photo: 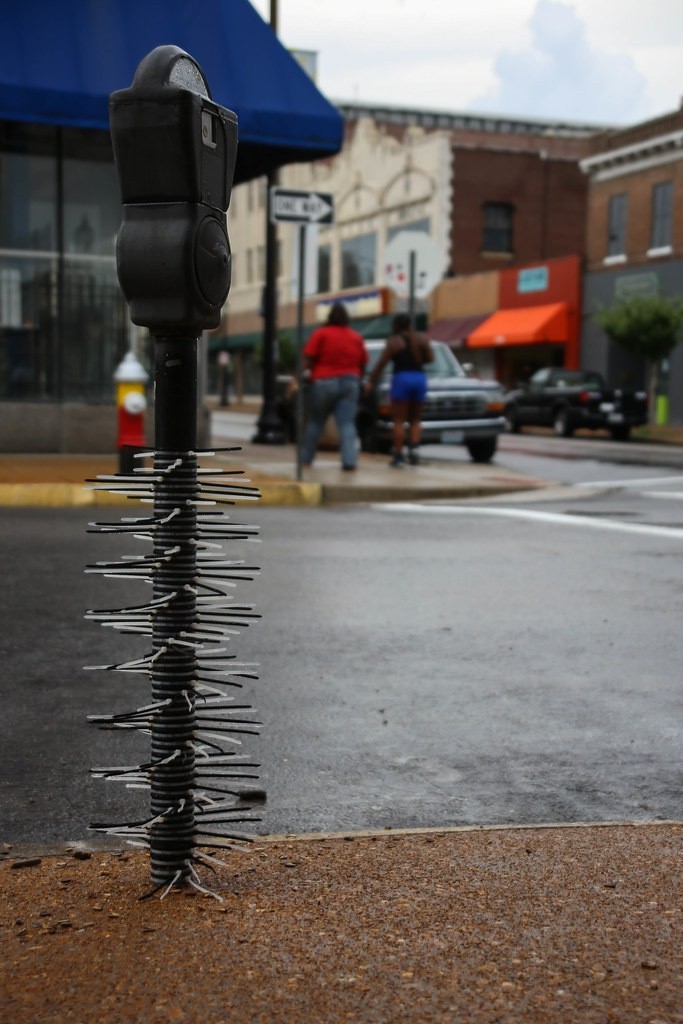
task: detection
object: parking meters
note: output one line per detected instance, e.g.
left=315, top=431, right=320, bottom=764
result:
left=109, top=43, right=241, bottom=893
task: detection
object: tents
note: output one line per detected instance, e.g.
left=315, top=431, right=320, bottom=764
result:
left=0, top=0, right=346, bottom=405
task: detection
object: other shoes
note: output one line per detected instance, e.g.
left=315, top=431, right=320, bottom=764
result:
left=341, top=467, right=356, bottom=482
left=409, top=451, right=421, bottom=464
left=300, top=464, right=312, bottom=481
left=389, top=455, right=405, bottom=469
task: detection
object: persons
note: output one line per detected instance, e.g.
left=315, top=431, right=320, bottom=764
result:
left=298, top=303, right=370, bottom=471
left=362, top=312, right=434, bottom=466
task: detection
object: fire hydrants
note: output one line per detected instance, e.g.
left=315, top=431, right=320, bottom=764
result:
left=112, top=350, right=151, bottom=478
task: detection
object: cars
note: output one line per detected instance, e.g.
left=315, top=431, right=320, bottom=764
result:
left=498, top=367, right=649, bottom=440
left=277, top=339, right=509, bottom=463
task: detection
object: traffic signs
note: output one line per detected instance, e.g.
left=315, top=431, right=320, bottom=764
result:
left=271, top=184, right=335, bottom=224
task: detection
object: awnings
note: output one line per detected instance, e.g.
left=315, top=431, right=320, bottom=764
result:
left=466, top=300, right=568, bottom=347
left=426, top=310, right=492, bottom=348
